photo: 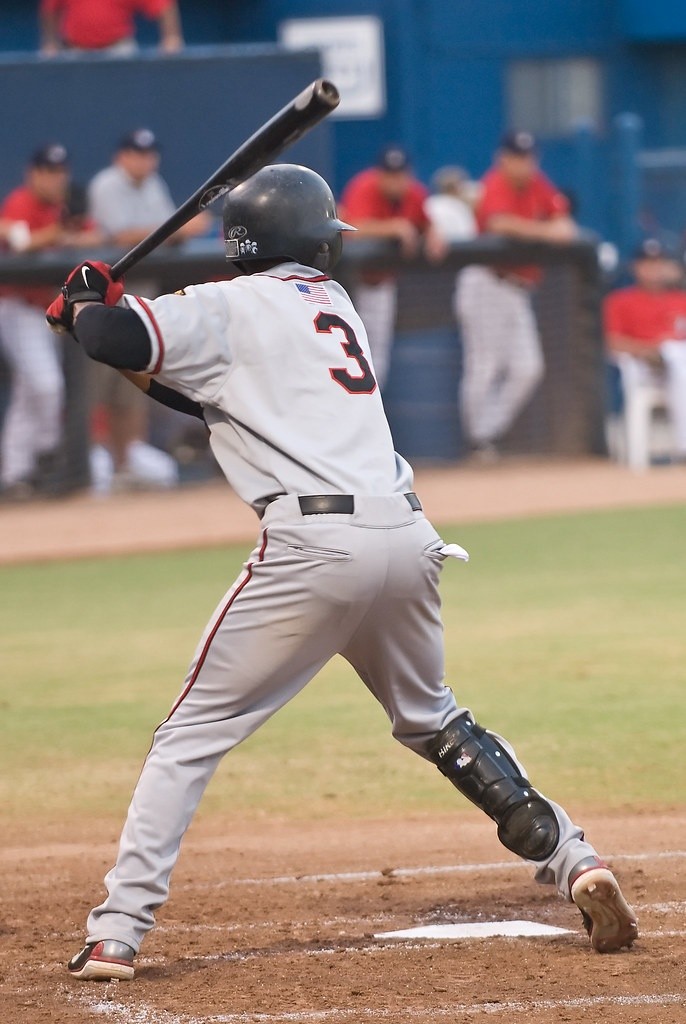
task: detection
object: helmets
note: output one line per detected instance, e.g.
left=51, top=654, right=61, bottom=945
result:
left=222, top=164, right=359, bottom=275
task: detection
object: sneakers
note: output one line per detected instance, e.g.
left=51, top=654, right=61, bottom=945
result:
left=68, top=939, right=135, bottom=980
left=568, top=855, right=638, bottom=953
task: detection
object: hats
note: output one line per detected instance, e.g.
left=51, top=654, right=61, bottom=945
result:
left=382, top=145, right=410, bottom=171
left=498, top=128, right=541, bottom=154
left=35, top=142, right=74, bottom=171
left=118, top=128, right=163, bottom=151
left=634, top=235, right=678, bottom=263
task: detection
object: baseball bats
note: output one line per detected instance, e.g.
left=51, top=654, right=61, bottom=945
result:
left=109, top=77, right=341, bottom=282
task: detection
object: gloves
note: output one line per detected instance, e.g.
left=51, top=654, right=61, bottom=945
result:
left=62, top=260, right=125, bottom=317
left=46, top=293, right=68, bottom=335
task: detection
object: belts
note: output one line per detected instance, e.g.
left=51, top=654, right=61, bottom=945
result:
left=298, top=492, right=423, bottom=516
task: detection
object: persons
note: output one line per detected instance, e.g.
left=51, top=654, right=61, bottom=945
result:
left=336, top=150, right=430, bottom=394
left=422, top=166, right=480, bottom=241
left=84, top=130, right=212, bottom=497
left=37, top=0, right=187, bottom=59
left=44, top=162, right=639, bottom=980
left=602, top=236, right=686, bottom=471
left=428, top=132, right=577, bottom=472
left=0, top=148, right=108, bottom=503
